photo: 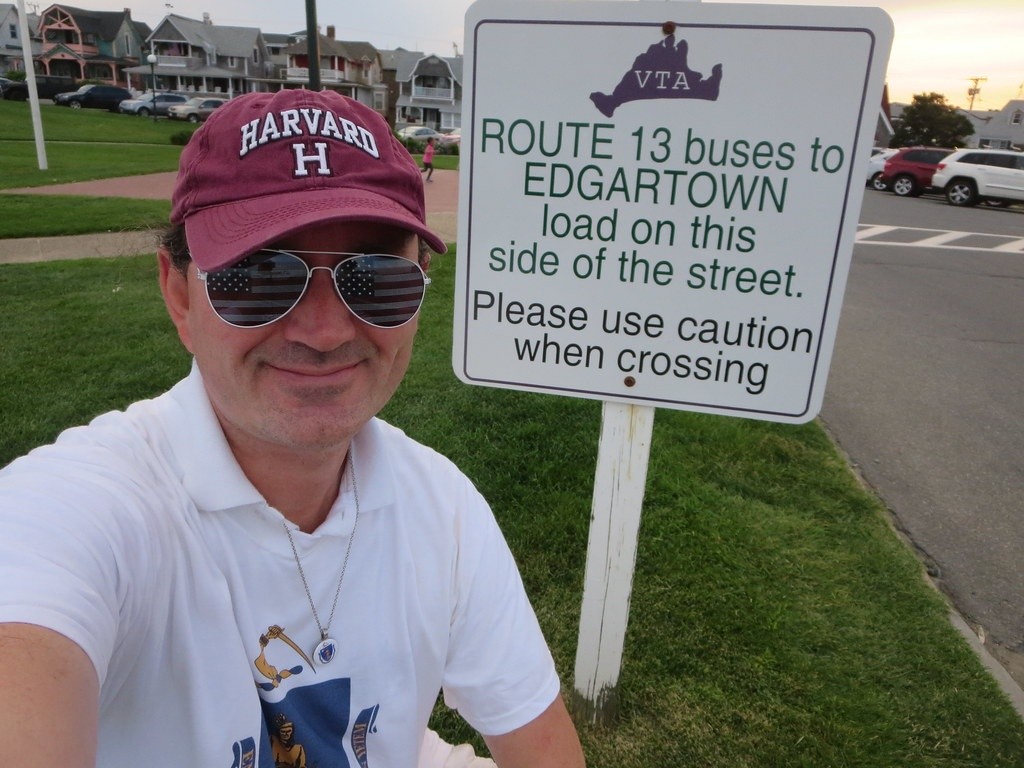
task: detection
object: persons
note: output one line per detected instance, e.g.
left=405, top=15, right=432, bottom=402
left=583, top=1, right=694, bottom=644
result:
left=420, top=137, right=436, bottom=182
left=2, top=91, right=589, bottom=768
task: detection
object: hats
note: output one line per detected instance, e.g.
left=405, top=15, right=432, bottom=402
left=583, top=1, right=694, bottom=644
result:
left=170, top=86, right=447, bottom=272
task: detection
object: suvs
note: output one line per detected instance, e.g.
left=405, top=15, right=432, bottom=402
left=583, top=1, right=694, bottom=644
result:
left=883, top=147, right=952, bottom=199
left=5, top=72, right=75, bottom=104
left=932, top=146, right=1024, bottom=210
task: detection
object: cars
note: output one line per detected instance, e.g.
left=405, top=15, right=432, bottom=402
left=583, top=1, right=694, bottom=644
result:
left=866, top=147, right=899, bottom=193
left=440, top=126, right=461, bottom=149
left=119, top=91, right=191, bottom=119
left=167, top=94, right=227, bottom=123
left=0, top=77, right=11, bottom=93
left=396, top=121, right=441, bottom=142
left=51, top=83, right=129, bottom=110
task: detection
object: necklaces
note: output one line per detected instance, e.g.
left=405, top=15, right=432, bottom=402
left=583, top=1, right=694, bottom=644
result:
left=277, top=445, right=360, bottom=663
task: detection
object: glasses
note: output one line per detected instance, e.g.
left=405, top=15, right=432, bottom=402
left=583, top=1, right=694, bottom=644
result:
left=196, top=248, right=432, bottom=329
